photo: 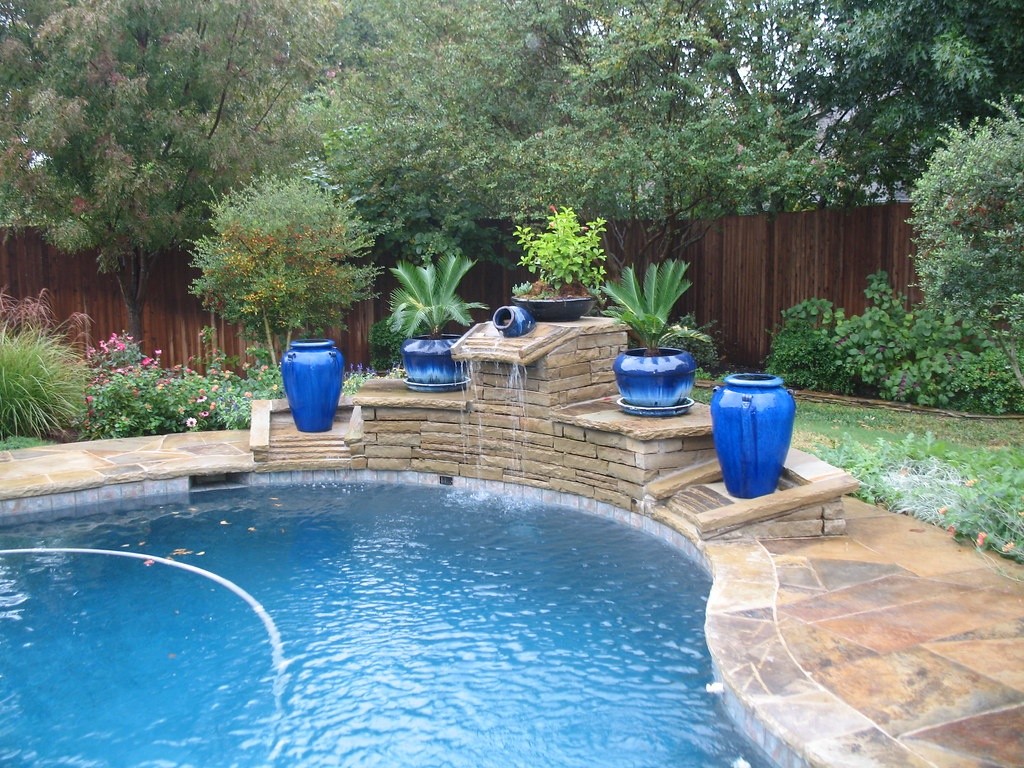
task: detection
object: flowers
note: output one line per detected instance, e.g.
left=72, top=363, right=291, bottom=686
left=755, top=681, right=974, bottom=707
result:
left=509, top=205, right=607, bottom=287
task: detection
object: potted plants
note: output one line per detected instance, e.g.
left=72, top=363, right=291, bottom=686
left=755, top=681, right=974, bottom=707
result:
left=510, top=281, right=597, bottom=322
left=596, top=260, right=711, bottom=419
left=386, top=253, right=472, bottom=392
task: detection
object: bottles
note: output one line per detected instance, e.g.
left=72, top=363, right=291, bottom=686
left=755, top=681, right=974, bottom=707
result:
left=492, top=305, right=534, bottom=338
left=281, top=338, right=345, bottom=433
left=710, top=373, right=796, bottom=499
